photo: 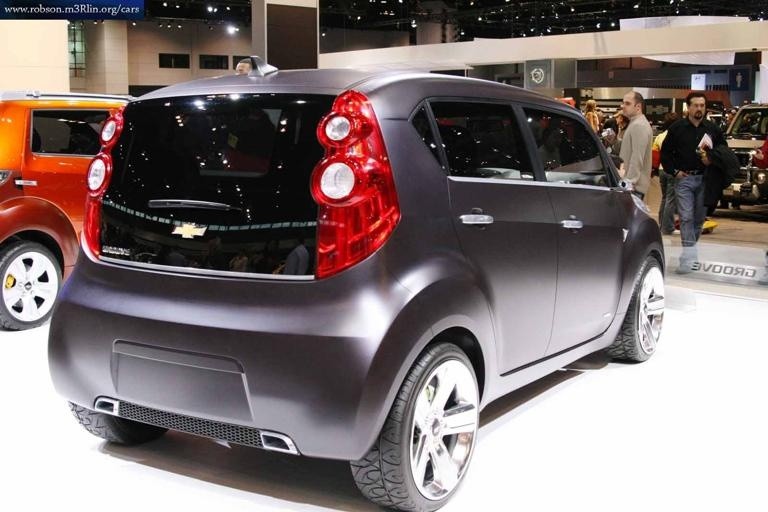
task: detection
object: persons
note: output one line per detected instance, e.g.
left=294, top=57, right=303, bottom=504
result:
left=100, top=221, right=312, bottom=276
left=233, top=56, right=253, bottom=77
left=538, top=125, right=565, bottom=169
left=581, top=89, right=757, bottom=276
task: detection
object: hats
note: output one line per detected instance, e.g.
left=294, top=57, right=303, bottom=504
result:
left=613, top=111, right=630, bottom=120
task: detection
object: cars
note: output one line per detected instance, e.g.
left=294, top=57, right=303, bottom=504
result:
left=1, top=97, right=278, bottom=330
left=48, top=54, right=668, bottom=512
left=706, top=114, right=728, bottom=128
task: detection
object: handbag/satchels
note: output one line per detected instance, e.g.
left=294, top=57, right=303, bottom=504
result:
left=651, top=144, right=661, bottom=170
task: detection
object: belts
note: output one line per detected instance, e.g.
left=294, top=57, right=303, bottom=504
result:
left=686, top=169, right=704, bottom=176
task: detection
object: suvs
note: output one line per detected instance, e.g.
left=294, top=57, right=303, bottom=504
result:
left=718, top=99, right=768, bottom=209
